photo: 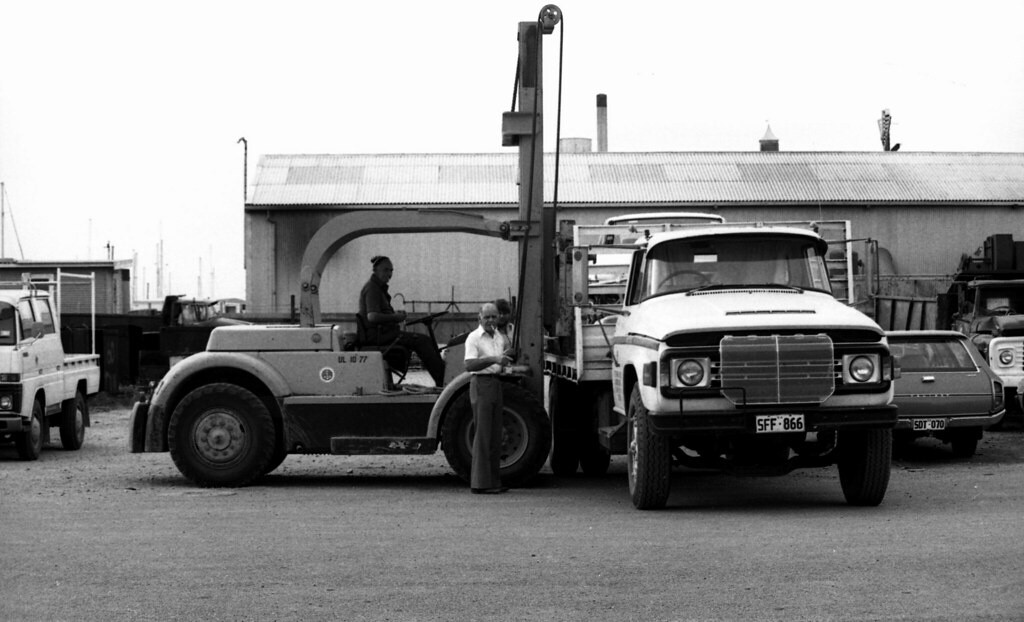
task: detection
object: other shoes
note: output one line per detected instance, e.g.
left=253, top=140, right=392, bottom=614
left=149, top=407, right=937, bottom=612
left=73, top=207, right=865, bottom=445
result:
left=471, top=486, right=511, bottom=494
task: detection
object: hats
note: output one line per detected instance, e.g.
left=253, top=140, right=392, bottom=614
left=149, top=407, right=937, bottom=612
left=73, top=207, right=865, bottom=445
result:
left=495, top=299, right=511, bottom=314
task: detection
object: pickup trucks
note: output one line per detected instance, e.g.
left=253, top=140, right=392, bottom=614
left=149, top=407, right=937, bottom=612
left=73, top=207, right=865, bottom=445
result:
left=0, top=288, right=103, bottom=459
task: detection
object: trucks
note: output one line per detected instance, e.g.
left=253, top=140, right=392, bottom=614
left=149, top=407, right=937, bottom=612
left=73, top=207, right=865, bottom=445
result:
left=550, top=209, right=902, bottom=508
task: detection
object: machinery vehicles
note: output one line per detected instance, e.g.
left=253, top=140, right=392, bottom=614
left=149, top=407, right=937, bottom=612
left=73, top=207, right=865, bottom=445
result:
left=129, top=3, right=559, bottom=490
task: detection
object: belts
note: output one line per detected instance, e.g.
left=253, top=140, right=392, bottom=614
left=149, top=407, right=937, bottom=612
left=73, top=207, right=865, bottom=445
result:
left=472, top=373, right=500, bottom=378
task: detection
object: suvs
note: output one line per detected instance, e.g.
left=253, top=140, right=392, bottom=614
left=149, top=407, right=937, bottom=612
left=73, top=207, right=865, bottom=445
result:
left=950, top=278, right=1024, bottom=429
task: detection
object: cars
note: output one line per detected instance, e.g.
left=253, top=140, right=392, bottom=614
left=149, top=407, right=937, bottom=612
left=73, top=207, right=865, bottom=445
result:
left=881, top=327, right=1007, bottom=460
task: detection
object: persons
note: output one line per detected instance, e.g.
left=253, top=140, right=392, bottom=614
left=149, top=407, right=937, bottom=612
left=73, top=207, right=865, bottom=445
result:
left=950, top=300, right=975, bottom=337
left=464, top=299, right=516, bottom=494
left=358, top=255, right=447, bottom=386
